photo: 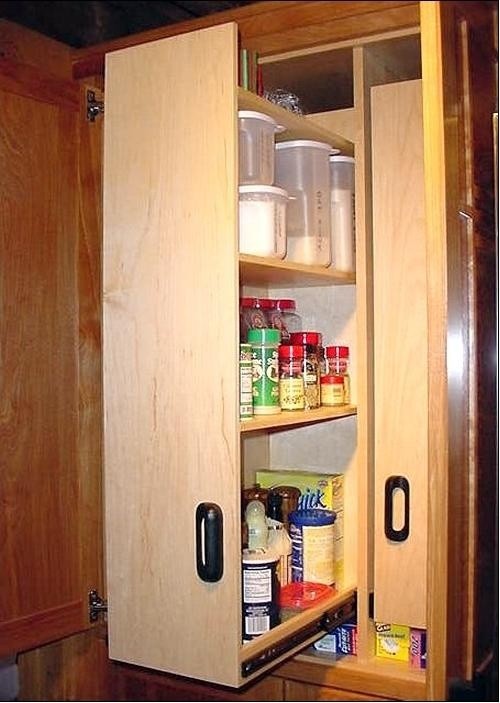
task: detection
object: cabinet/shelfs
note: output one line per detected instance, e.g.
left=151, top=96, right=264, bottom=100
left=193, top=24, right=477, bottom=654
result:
left=0, top=0, right=499, bottom=702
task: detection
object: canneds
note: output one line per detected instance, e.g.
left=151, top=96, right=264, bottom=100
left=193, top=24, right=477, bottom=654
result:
left=240, top=297, right=350, bottom=419
left=287, top=508, right=337, bottom=588
left=242, top=554, right=280, bottom=641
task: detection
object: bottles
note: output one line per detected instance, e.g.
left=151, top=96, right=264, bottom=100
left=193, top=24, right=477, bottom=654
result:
left=243, top=492, right=283, bottom=553
left=241, top=298, right=352, bottom=420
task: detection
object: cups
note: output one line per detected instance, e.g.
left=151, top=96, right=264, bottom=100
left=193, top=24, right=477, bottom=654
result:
left=239, top=110, right=356, bottom=273
